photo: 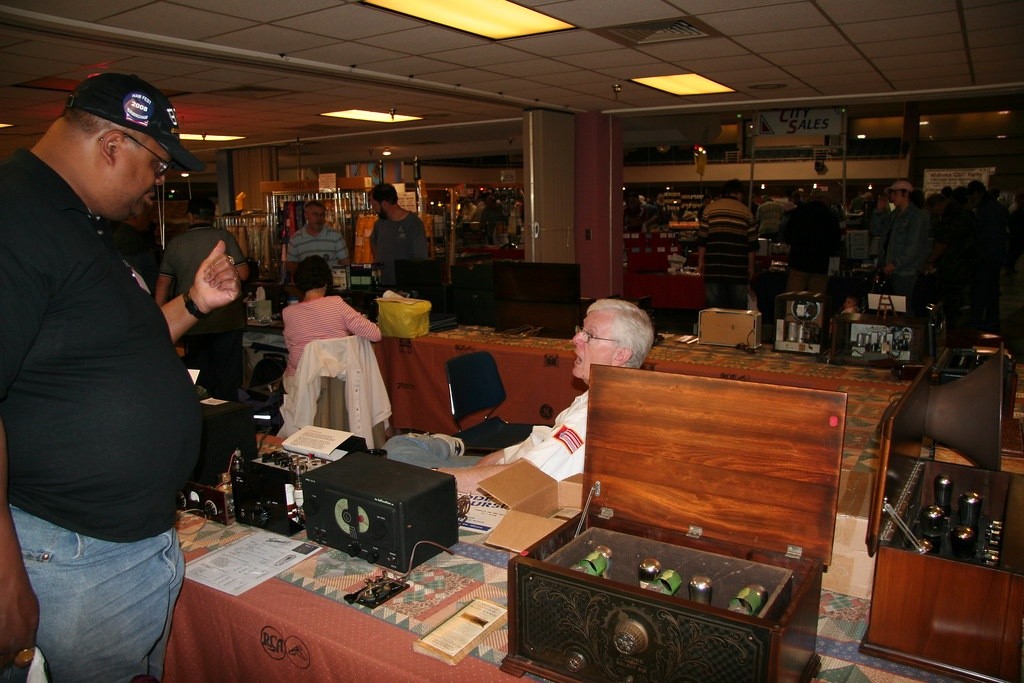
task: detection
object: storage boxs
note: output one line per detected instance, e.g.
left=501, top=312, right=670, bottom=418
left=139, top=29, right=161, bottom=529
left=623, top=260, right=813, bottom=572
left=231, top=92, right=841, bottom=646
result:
left=866, top=357, right=1024, bottom=683
left=476, top=461, right=584, bottom=559
left=259, top=176, right=372, bottom=193
left=498, top=364, right=849, bottom=683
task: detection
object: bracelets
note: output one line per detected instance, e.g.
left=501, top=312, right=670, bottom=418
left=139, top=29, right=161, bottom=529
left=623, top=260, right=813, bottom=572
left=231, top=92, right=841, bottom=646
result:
left=430, top=466, right=438, bottom=471
left=181, top=290, right=212, bottom=320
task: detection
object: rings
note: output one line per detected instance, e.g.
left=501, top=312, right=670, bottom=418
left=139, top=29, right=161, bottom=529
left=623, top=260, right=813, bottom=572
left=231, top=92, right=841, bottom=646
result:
left=233, top=268, right=240, bottom=283
left=228, top=255, right=235, bottom=265
left=14, top=647, right=34, bottom=669
left=0, top=72, right=240, bottom=683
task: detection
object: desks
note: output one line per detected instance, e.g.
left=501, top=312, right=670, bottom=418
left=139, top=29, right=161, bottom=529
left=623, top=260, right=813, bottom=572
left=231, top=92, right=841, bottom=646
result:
left=277, top=336, right=394, bottom=450
left=623, top=268, right=706, bottom=309
left=162, top=324, right=1024, bottom=682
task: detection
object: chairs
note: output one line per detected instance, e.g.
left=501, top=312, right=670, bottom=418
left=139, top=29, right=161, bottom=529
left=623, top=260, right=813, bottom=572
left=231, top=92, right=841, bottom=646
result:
left=443, top=350, right=553, bottom=456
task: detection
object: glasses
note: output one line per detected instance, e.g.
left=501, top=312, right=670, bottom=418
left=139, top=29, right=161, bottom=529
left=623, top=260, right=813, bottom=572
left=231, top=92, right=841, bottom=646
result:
left=98, top=133, right=173, bottom=177
left=575, top=325, right=616, bottom=343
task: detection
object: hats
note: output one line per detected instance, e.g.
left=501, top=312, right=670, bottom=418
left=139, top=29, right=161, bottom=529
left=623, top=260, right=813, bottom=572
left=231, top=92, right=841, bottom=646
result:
left=887, top=181, right=913, bottom=192
left=182, top=197, right=215, bottom=219
left=65, top=74, right=208, bottom=171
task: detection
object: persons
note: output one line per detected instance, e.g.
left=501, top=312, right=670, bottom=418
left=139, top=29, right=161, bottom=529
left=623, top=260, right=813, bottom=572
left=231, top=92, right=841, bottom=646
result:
left=381, top=298, right=654, bottom=496
left=368, top=183, right=430, bottom=285
left=111, top=193, right=249, bottom=398
left=695, top=179, right=1024, bottom=368
left=623, top=193, right=697, bottom=231
left=275, top=255, right=382, bottom=413
left=285, top=200, right=350, bottom=297
left=458, top=192, right=524, bottom=245
